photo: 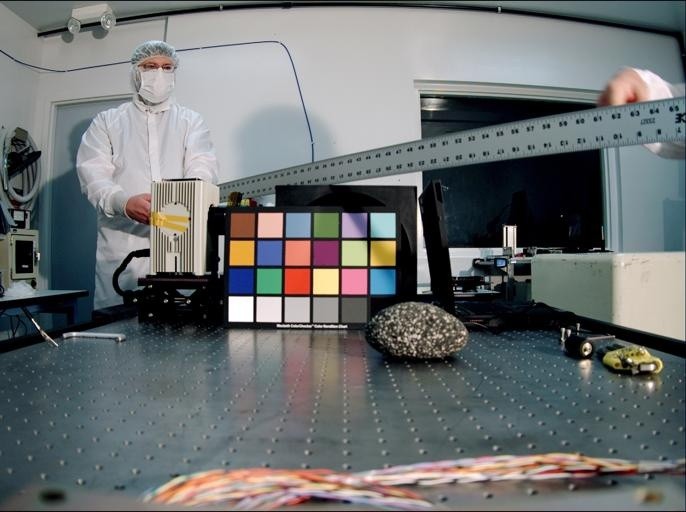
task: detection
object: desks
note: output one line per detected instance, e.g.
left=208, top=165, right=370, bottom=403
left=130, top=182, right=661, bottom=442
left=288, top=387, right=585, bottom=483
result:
left=0, top=290, right=89, bottom=326
left=474, top=256, right=531, bottom=304
left=417, top=286, right=502, bottom=300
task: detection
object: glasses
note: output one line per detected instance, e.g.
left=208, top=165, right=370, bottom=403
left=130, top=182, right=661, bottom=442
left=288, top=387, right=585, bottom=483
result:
left=138, top=63, right=174, bottom=72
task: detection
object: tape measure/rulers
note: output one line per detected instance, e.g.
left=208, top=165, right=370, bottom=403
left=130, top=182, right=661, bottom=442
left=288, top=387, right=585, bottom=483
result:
left=218, top=97, right=686, bottom=203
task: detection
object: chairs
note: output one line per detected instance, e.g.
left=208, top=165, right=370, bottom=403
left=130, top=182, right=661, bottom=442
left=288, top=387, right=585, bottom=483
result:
left=418, top=178, right=575, bottom=331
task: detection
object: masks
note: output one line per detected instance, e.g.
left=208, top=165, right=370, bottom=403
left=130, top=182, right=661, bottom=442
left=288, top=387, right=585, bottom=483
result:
left=138, top=69, right=174, bottom=104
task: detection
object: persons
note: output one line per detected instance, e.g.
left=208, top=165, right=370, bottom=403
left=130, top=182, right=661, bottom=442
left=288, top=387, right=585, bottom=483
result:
left=77, top=40, right=220, bottom=309
left=597, top=65, right=685, bottom=159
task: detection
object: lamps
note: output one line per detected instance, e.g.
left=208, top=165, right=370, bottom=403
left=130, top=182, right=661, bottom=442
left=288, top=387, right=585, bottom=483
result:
left=67, top=4, right=116, bottom=34
